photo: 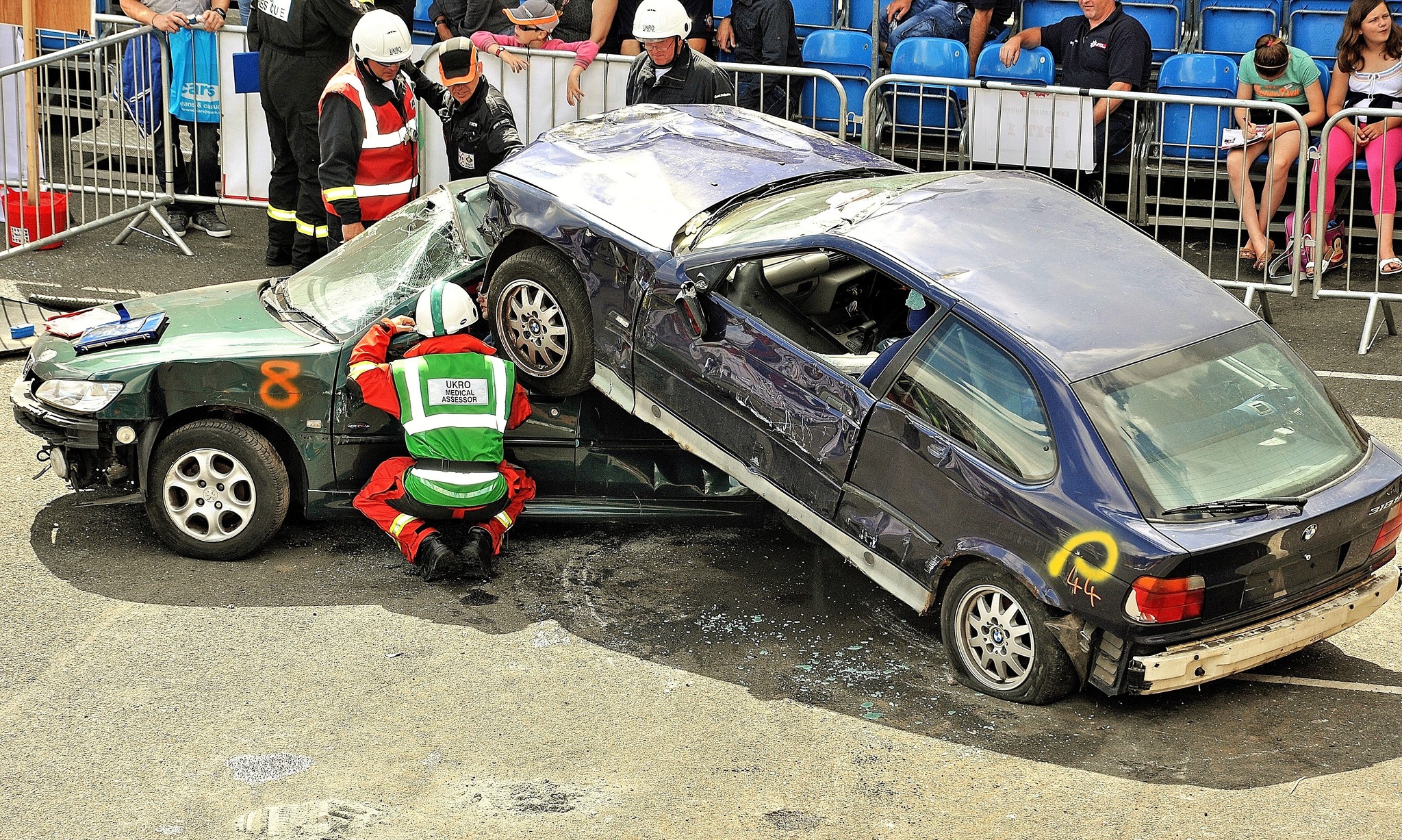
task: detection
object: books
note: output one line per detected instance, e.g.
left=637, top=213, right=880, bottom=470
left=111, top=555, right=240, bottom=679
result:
left=233, top=52, right=261, bottom=94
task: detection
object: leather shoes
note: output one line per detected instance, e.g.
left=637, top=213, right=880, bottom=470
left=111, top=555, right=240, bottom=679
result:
left=459, top=527, right=491, bottom=580
left=418, top=536, right=454, bottom=583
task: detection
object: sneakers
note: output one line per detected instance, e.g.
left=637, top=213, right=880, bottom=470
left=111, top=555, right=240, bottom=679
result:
left=189, top=210, right=232, bottom=237
left=162, top=212, right=189, bottom=235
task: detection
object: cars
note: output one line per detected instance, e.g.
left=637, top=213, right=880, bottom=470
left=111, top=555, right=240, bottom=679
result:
left=485, top=99, right=1402, bottom=706
left=10, top=173, right=827, bottom=560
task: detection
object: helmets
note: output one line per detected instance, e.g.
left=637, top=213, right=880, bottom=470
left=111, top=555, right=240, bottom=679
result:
left=351, top=7, right=411, bottom=62
left=632, top=0, right=693, bottom=41
left=416, top=280, right=477, bottom=338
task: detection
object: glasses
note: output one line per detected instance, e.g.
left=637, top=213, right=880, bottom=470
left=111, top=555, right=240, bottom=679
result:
left=639, top=38, right=675, bottom=52
left=378, top=57, right=410, bottom=67
left=518, top=24, right=541, bottom=31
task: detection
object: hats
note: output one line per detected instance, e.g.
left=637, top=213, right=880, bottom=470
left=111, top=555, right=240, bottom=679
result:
left=437, top=35, right=477, bottom=87
left=503, top=0, right=560, bottom=33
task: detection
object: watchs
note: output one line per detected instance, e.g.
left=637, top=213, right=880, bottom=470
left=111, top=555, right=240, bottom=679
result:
left=495, top=46, right=505, bottom=58
left=434, top=20, right=445, bottom=27
left=213, top=8, right=226, bottom=21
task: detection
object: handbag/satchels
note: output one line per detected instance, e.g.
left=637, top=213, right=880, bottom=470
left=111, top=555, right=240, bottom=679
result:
left=1267, top=207, right=1350, bottom=283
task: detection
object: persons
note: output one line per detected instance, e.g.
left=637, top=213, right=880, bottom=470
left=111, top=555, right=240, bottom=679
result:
left=866, top=0, right=1013, bottom=82
left=374, top=0, right=417, bottom=36
left=476, top=277, right=496, bottom=347
left=428, top=0, right=520, bottom=51
left=1226, top=34, right=1326, bottom=268
left=246, top=1, right=376, bottom=276
left=626, top=0, right=737, bottom=109
left=716, top=0, right=805, bottom=120
left=999, top=0, right=1152, bottom=208
left=317, top=10, right=418, bottom=250
left=549, top=0, right=618, bottom=52
left=620, top=0, right=714, bottom=62
left=1306, top=0, right=1402, bottom=281
left=470, top=0, right=599, bottom=106
left=400, top=36, right=525, bottom=182
left=347, top=281, right=536, bottom=583
left=113, top=0, right=233, bottom=238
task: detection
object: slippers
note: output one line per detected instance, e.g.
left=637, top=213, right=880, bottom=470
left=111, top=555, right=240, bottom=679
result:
left=1306, top=243, right=1335, bottom=281
left=1377, top=258, right=1402, bottom=274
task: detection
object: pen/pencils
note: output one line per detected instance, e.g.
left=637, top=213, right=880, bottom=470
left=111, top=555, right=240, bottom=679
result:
left=1242, top=116, right=1258, bottom=138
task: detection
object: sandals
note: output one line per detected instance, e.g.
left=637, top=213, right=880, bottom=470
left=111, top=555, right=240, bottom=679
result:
left=1238, top=245, right=1258, bottom=262
left=1253, top=235, right=1275, bottom=270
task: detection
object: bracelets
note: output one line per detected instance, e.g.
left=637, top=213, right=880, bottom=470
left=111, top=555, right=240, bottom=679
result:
left=151, top=13, right=161, bottom=26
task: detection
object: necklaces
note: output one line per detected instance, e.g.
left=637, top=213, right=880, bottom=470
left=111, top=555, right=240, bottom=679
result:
left=556, top=0, right=571, bottom=16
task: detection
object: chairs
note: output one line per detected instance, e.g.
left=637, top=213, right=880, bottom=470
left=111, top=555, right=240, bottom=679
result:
left=414, top=0, right=1402, bottom=167
